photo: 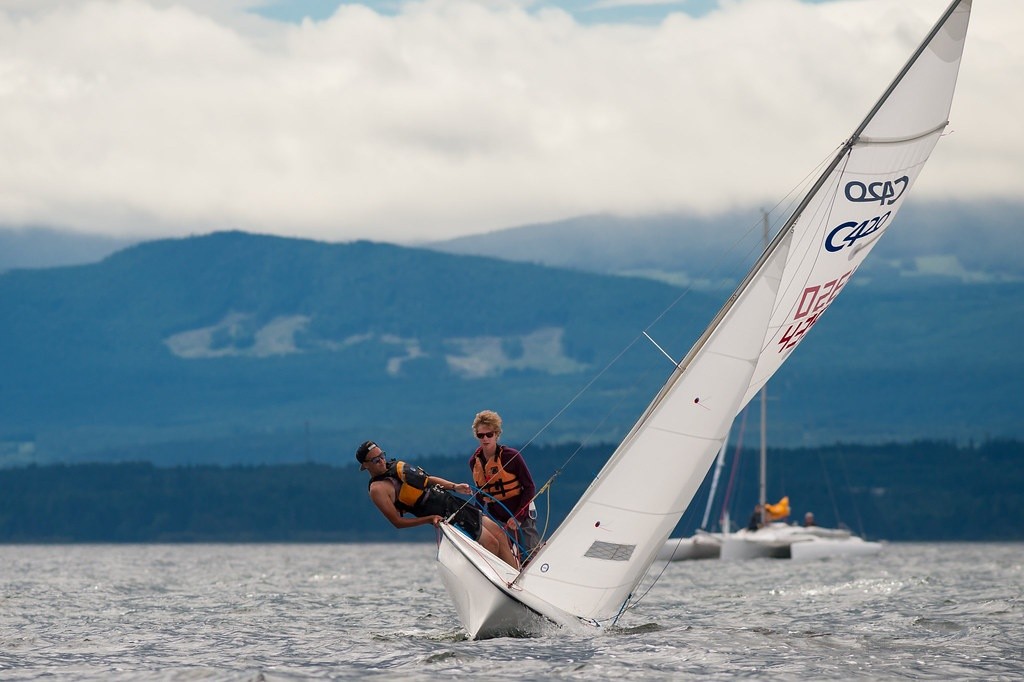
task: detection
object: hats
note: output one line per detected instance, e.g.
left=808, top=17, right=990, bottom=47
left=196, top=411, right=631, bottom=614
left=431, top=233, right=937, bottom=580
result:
left=355, top=441, right=378, bottom=471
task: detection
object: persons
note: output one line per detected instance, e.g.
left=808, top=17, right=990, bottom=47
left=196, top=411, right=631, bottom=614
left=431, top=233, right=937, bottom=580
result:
left=470, top=410, right=541, bottom=571
left=802, top=513, right=816, bottom=528
left=357, top=441, right=519, bottom=572
left=748, top=504, right=763, bottom=532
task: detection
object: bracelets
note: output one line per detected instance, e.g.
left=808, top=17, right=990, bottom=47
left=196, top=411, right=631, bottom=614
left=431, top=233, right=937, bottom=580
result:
left=452, top=483, right=456, bottom=492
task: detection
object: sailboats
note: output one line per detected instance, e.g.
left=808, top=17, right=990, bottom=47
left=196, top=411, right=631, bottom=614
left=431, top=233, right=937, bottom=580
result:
left=646, top=205, right=884, bottom=562
left=432, top=0, right=970, bottom=647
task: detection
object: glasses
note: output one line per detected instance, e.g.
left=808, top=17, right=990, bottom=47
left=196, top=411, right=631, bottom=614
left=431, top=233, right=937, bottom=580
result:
left=363, top=451, right=386, bottom=464
left=477, top=431, right=495, bottom=439
